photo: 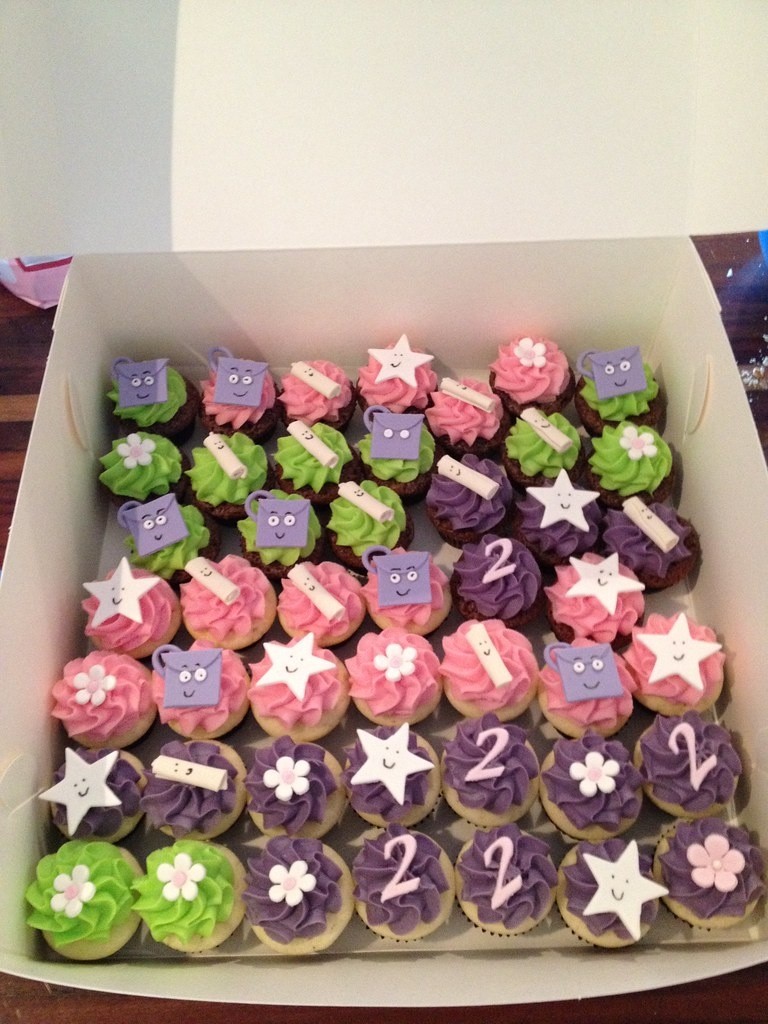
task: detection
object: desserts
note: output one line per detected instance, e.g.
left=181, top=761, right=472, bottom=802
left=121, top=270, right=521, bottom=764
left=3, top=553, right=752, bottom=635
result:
left=25, top=334, right=768, bottom=948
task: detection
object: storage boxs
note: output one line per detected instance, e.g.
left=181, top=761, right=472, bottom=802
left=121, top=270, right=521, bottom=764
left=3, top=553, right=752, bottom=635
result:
left=0, top=232, right=767, bottom=1022
